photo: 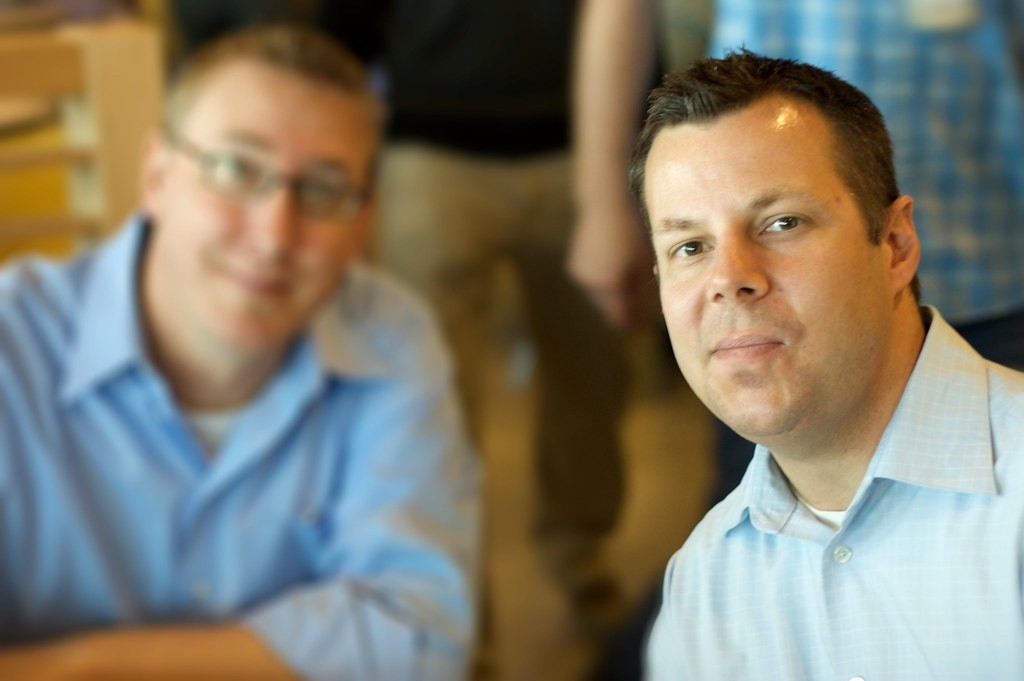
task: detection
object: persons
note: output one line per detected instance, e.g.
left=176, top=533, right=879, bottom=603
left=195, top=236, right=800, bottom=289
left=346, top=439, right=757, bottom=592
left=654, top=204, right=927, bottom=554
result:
left=163, top=0, right=1024, bottom=681
left=0, top=27, right=487, bottom=681
left=631, top=51, right=1024, bottom=681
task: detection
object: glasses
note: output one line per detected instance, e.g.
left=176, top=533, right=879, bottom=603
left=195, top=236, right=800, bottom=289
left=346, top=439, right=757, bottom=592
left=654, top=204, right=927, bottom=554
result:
left=164, top=131, right=370, bottom=219
